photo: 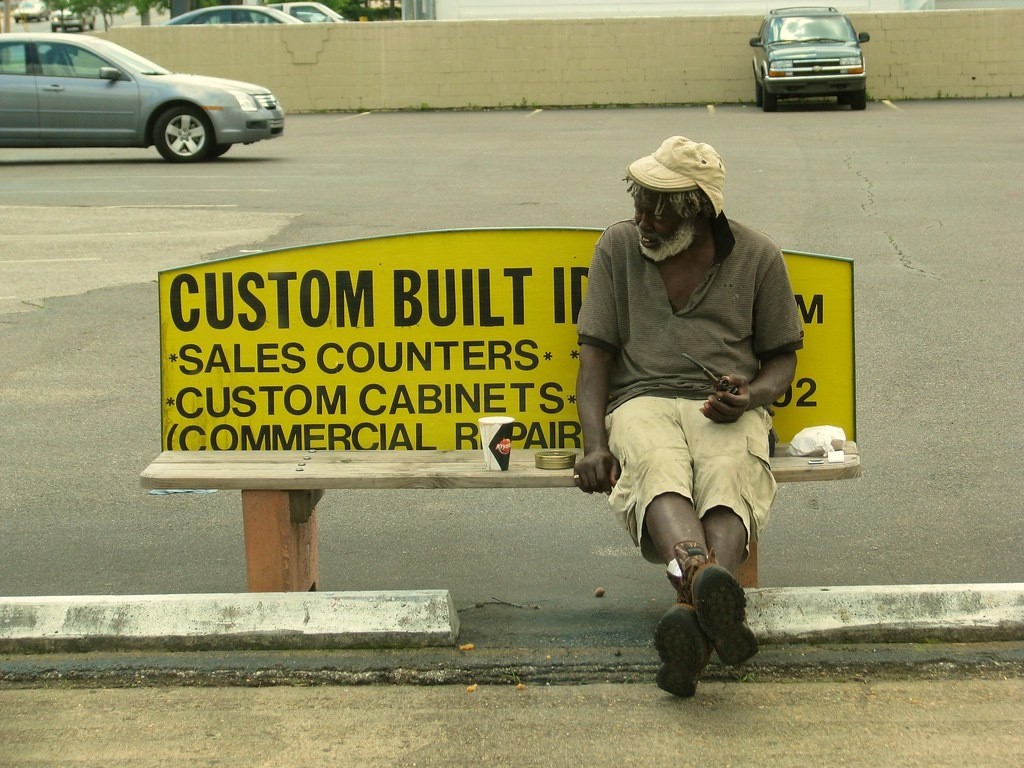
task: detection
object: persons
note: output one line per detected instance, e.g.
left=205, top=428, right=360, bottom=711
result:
left=573, top=135, right=804, bottom=699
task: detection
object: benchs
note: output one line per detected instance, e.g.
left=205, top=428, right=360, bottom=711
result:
left=140, top=228, right=863, bottom=593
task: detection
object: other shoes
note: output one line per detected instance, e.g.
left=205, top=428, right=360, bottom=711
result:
left=654, top=540, right=759, bottom=697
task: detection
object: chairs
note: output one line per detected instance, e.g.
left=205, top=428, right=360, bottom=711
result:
left=39, top=50, right=69, bottom=76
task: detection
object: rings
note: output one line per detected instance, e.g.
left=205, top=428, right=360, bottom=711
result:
left=573, top=474, right=579, bottom=478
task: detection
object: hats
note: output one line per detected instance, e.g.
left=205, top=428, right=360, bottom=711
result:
left=629, top=135, right=726, bottom=220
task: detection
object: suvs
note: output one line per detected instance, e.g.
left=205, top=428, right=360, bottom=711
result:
left=50, top=2, right=95, bottom=32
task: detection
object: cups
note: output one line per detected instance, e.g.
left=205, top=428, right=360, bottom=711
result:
left=478, top=417, right=515, bottom=471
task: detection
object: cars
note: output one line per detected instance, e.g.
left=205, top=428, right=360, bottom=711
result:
left=748, top=6, right=870, bottom=112
left=0, top=32, right=284, bottom=163
left=162, top=5, right=304, bottom=26
left=269, top=2, right=348, bottom=23
left=11, top=0, right=50, bottom=23
